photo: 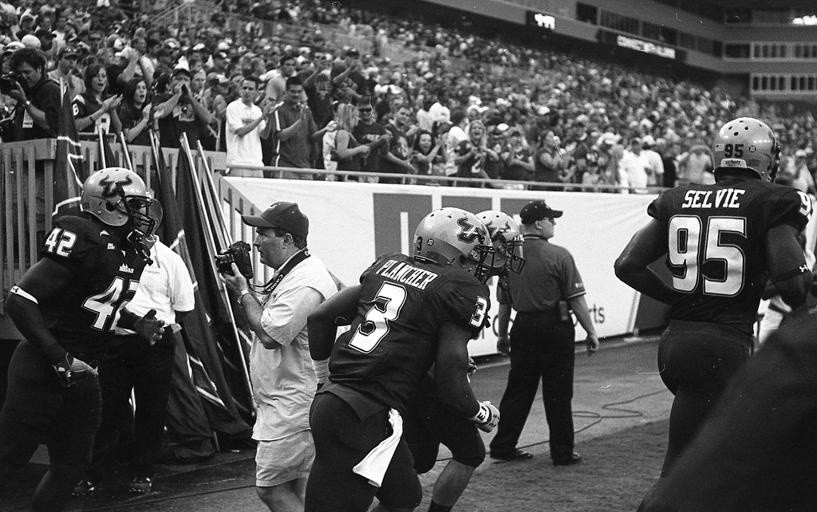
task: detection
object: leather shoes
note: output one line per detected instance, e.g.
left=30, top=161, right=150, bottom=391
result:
left=571, top=452, right=581, bottom=462
left=72, top=480, right=97, bottom=498
left=128, top=477, right=153, bottom=494
left=490, top=448, right=534, bottom=460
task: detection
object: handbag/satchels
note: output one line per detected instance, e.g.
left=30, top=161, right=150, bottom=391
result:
left=331, top=149, right=352, bottom=161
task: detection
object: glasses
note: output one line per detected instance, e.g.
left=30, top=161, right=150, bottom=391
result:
left=358, top=107, right=372, bottom=111
left=3, top=46, right=18, bottom=50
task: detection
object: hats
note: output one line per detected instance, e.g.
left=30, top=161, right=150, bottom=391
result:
left=5, top=41, right=25, bottom=54
left=604, top=111, right=660, bottom=146
left=520, top=199, right=563, bottom=224
left=241, top=201, right=309, bottom=238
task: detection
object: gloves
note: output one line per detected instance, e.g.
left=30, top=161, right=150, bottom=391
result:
left=475, top=400, right=501, bottom=433
left=69, top=357, right=100, bottom=385
left=132, top=309, right=166, bottom=347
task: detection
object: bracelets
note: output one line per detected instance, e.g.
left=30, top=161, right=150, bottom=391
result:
left=237, top=289, right=251, bottom=304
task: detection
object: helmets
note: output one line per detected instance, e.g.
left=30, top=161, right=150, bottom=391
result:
left=712, top=117, right=781, bottom=183
left=78, top=166, right=162, bottom=251
left=412, top=206, right=497, bottom=285
left=475, top=209, right=528, bottom=275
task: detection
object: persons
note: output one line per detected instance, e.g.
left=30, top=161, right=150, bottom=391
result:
left=304, top=206, right=500, bottom=511
left=2, top=168, right=165, bottom=512
left=1, top=4, right=817, bottom=196
left=405, top=210, right=527, bottom=510
left=71, top=194, right=194, bottom=495
left=489, top=199, right=601, bottom=466
left=638, top=302, right=816, bottom=511
left=215, top=201, right=350, bottom=512
left=613, top=115, right=817, bottom=478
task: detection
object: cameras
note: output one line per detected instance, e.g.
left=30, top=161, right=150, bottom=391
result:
left=214, top=241, right=253, bottom=280
left=0, top=71, right=29, bottom=96
